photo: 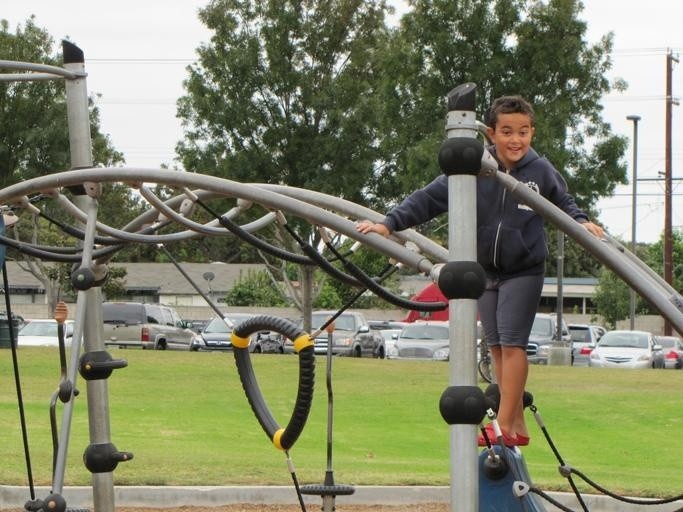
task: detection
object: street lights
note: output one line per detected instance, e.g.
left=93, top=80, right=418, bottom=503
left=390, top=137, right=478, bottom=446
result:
left=625, top=114, right=643, bottom=332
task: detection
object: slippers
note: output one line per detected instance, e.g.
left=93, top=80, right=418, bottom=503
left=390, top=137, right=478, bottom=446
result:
left=516, top=432, right=530, bottom=445
left=478, top=423, right=518, bottom=446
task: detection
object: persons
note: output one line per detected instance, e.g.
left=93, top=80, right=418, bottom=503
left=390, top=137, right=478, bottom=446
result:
left=353, top=92, right=606, bottom=448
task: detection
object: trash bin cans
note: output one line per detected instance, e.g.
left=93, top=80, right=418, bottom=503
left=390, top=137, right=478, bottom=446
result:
left=0, top=319, right=19, bottom=348
